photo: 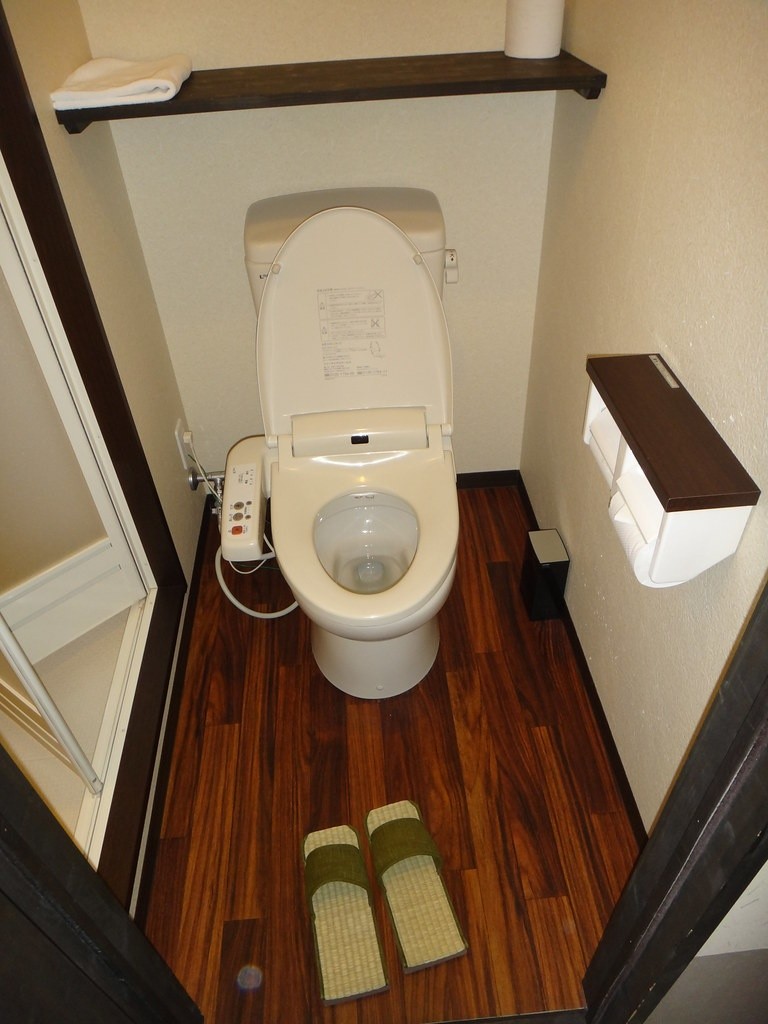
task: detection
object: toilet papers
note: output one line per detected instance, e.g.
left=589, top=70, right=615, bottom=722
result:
left=588, top=407, right=690, bottom=588
left=504, top=0, right=565, bottom=59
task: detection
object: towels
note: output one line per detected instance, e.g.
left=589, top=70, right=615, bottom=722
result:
left=50, top=54, right=193, bottom=111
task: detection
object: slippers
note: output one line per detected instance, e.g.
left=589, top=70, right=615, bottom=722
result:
left=362, top=800, right=469, bottom=976
left=303, top=824, right=389, bottom=1002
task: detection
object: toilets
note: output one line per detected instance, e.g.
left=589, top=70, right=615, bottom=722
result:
left=220, top=185, right=461, bottom=701
